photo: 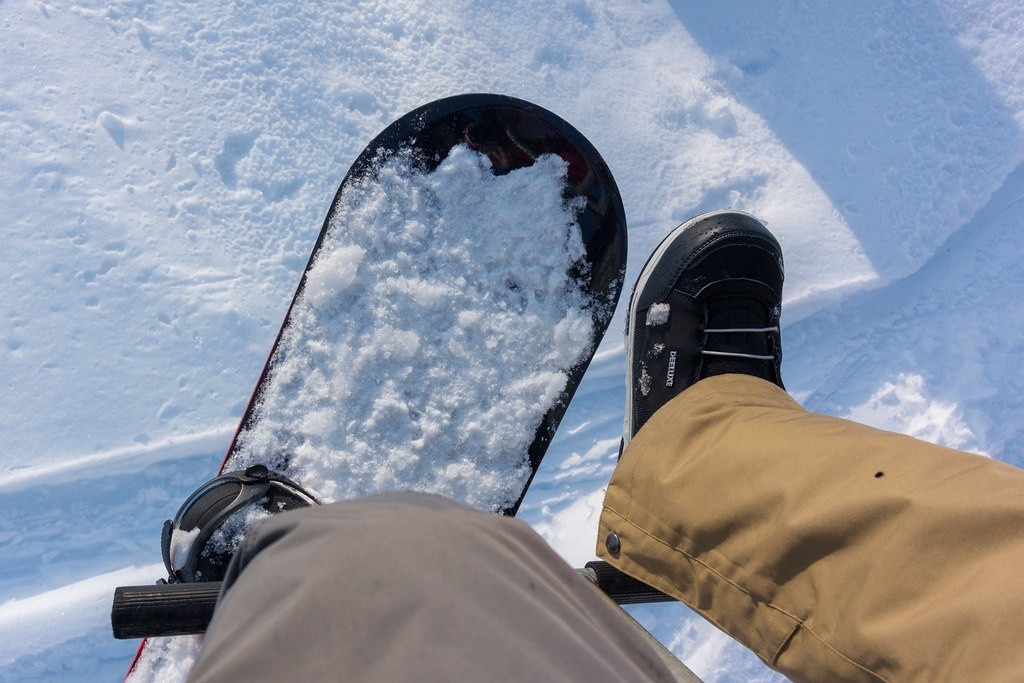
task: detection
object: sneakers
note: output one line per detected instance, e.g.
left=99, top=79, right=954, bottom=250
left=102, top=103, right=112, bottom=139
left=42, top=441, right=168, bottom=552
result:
left=167, top=473, right=320, bottom=583
left=624, top=207, right=785, bottom=450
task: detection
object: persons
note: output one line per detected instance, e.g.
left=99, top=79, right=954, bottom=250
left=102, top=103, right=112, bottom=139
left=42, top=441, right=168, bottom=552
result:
left=160, top=209, right=1024, bottom=683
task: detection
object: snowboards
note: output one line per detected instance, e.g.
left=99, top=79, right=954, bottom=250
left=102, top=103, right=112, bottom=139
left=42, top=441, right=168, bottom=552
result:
left=120, top=92, right=629, bottom=683
